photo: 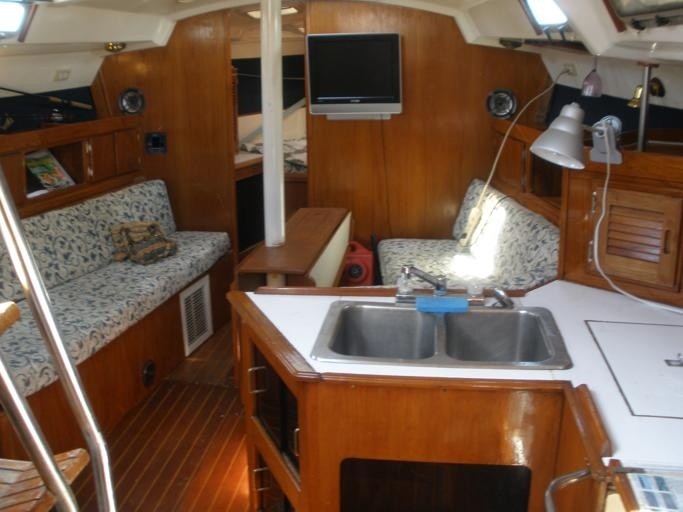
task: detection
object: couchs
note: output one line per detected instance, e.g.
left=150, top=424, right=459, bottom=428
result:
left=0, top=177, right=226, bottom=461
left=381, top=178, right=559, bottom=289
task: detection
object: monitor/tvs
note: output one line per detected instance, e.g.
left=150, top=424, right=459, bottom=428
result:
left=305, top=32, right=403, bottom=120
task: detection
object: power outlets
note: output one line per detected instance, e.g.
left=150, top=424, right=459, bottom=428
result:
left=564, top=64, right=576, bottom=75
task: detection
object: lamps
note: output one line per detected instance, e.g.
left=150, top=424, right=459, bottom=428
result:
left=531, top=103, right=624, bottom=172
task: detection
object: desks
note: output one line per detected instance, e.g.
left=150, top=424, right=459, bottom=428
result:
left=234, top=148, right=307, bottom=222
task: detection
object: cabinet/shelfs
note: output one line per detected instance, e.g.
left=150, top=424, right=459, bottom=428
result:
left=0, top=111, right=143, bottom=220
left=491, top=121, right=682, bottom=306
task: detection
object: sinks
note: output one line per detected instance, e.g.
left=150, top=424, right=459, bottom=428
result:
left=310, top=299, right=441, bottom=368
left=442, top=305, right=573, bottom=370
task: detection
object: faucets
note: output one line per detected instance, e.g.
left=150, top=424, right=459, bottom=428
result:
left=402, top=261, right=449, bottom=295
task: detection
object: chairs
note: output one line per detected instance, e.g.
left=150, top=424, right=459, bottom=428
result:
left=0, top=300, right=89, bottom=512
